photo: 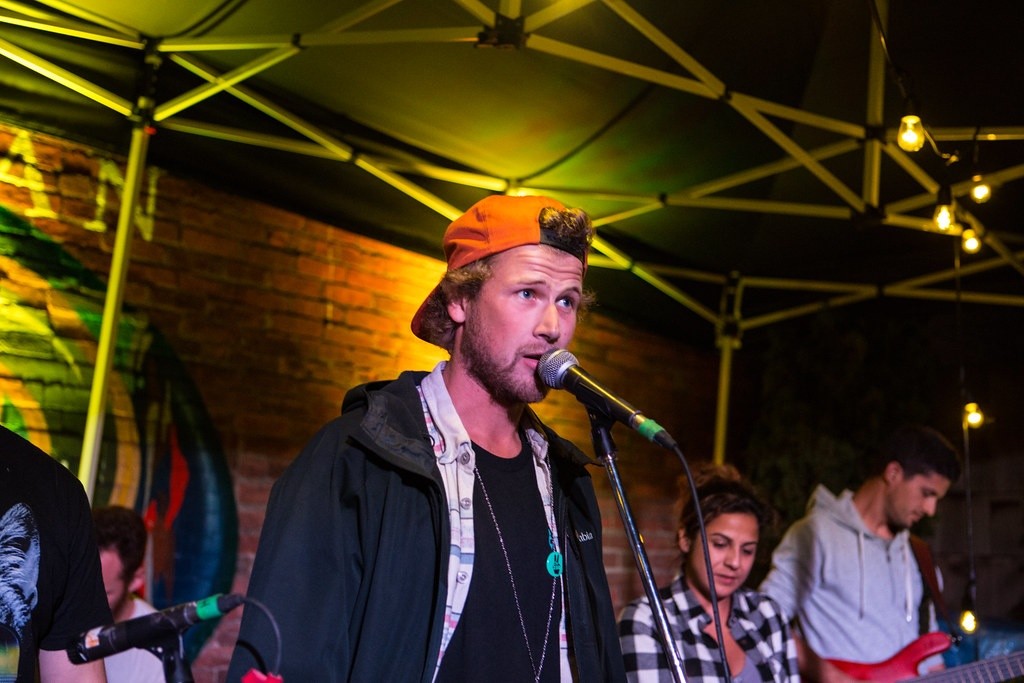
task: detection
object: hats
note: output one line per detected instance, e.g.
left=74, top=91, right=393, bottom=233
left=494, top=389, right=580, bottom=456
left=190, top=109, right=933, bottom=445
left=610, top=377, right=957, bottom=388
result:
left=411, top=196, right=587, bottom=352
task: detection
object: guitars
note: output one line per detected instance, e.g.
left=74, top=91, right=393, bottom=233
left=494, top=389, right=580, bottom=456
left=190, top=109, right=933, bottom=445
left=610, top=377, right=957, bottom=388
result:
left=820, top=631, right=1024, bottom=683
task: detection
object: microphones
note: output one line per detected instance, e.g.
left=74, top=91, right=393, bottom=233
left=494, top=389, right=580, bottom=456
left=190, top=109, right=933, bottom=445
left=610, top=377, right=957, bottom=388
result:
left=65, top=592, right=241, bottom=665
left=536, top=347, right=678, bottom=449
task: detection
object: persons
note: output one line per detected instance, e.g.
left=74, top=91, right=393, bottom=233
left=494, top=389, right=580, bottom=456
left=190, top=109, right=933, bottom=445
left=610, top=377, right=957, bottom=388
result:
left=757, top=426, right=952, bottom=683
left=617, top=466, right=800, bottom=683
left=91, top=504, right=184, bottom=683
left=0, top=424, right=115, bottom=683
left=226, top=194, right=629, bottom=683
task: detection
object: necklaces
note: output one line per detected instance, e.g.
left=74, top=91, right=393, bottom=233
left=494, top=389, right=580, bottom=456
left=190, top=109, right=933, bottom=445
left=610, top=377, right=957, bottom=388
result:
left=474, top=451, right=563, bottom=683
